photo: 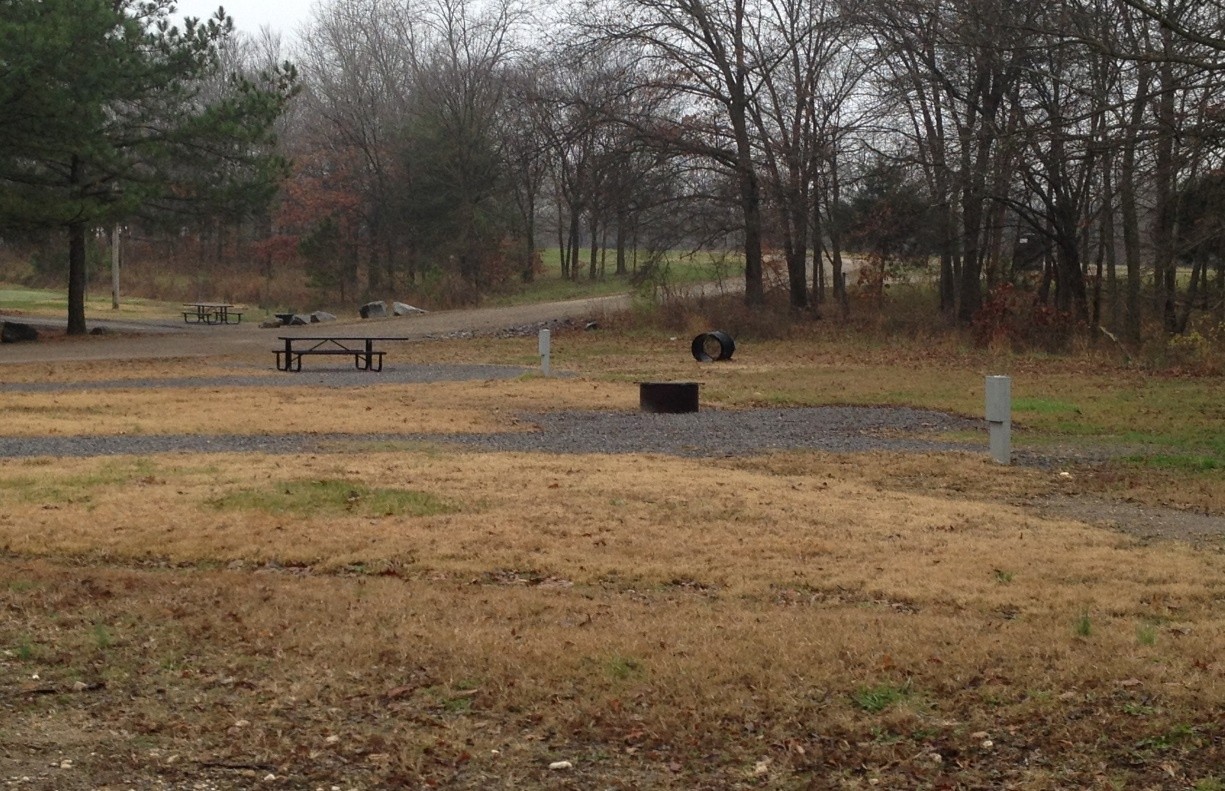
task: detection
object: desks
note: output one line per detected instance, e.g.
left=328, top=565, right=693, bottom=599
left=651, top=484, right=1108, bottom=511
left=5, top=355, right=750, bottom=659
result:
left=277, top=336, right=410, bottom=372
left=183, top=303, right=233, bottom=324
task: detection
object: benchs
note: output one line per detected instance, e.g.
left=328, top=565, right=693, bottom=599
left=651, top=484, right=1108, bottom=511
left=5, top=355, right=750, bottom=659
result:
left=271, top=349, right=365, bottom=354
left=213, top=311, right=243, bottom=315
left=291, top=349, right=388, bottom=355
left=180, top=312, right=212, bottom=315
